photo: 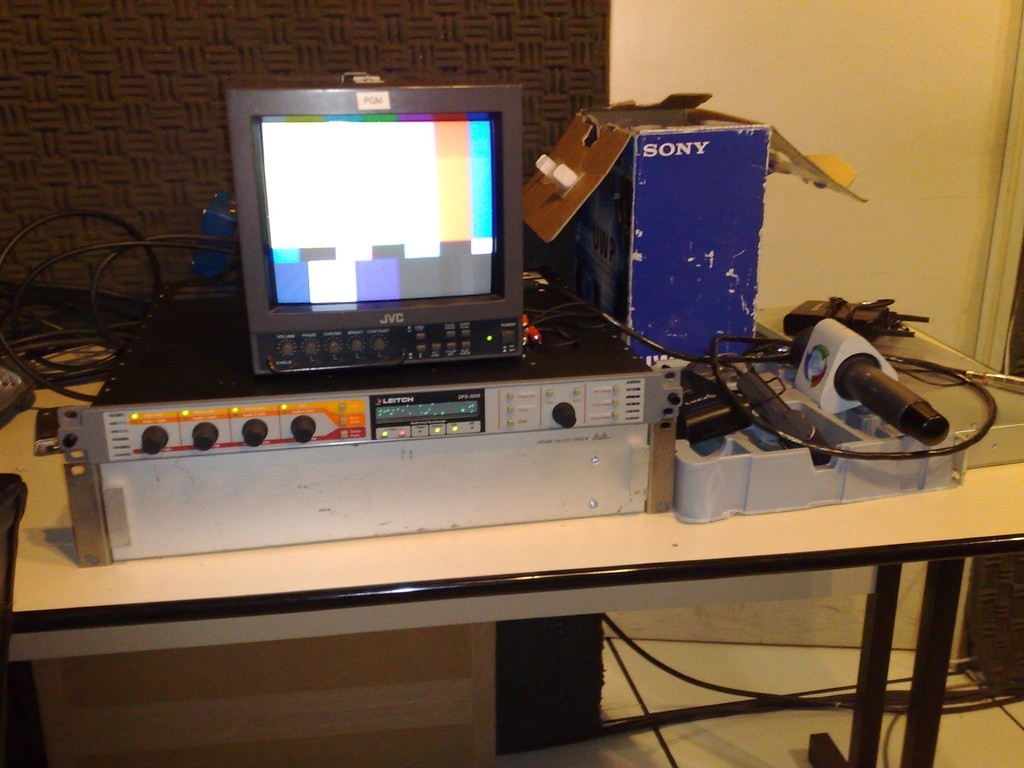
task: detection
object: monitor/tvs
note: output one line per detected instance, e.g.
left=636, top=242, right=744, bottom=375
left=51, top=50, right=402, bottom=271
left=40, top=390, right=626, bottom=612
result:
left=224, top=78, right=524, bottom=373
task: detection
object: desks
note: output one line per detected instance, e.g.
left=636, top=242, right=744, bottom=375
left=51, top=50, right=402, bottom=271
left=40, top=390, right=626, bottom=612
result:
left=1, top=325, right=1024, bottom=768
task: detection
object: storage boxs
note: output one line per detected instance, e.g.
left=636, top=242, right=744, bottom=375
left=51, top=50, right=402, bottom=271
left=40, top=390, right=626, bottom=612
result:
left=675, top=423, right=961, bottom=523
left=522, top=93, right=871, bottom=359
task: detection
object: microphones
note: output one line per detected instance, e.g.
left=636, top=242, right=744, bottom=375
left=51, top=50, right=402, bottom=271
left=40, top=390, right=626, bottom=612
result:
left=790, top=318, right=950, bottom=446
